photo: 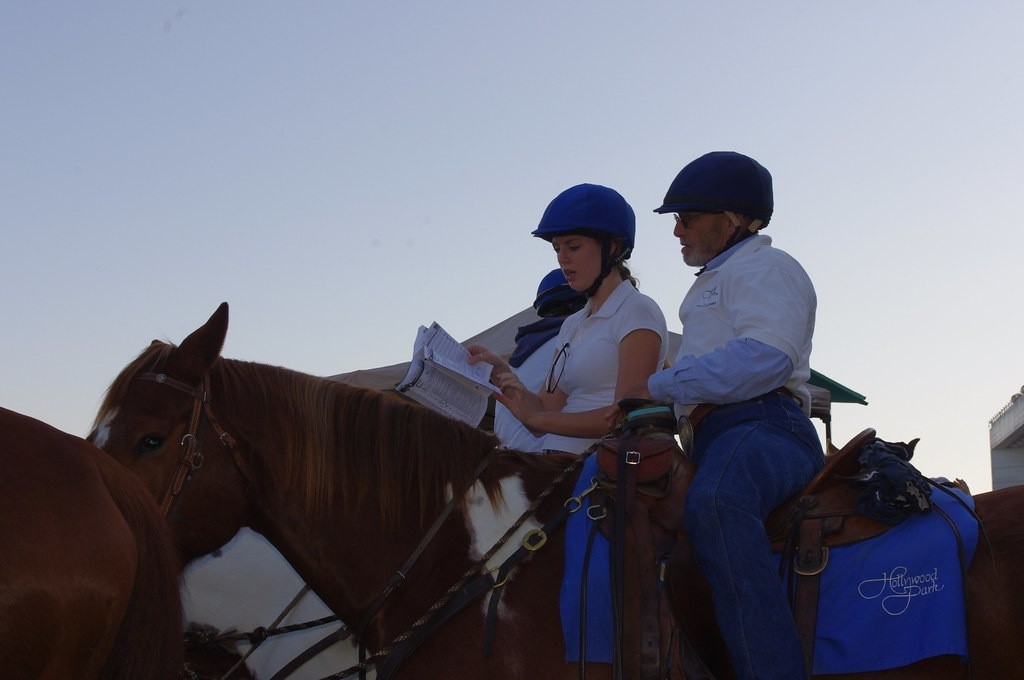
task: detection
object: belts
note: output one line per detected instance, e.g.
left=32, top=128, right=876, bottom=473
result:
left=688, top=385, right=792, bottom=430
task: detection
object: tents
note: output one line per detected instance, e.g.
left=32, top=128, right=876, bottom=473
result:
left=317, top=305, right=834, bottom=455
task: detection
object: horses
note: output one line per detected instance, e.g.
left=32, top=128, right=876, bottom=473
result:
left=0, top=299, right=1024, bottom=680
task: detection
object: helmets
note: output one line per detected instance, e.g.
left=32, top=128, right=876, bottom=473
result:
left=534, top=267, right=577, bottom=318
left=530, top=183, right=636, bottom=248
left=652, top=151, right=774, bottom=230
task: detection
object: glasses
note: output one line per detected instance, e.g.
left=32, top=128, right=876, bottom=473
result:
left=673, top=213, right=710, bottom=228
left=545, top=341, right=570, bottom=394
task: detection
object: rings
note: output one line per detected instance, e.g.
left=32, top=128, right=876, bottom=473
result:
left=479, top=353, right=483, bottom=361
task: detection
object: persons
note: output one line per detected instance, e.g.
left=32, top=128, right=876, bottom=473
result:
left=467, top=184, right=669, bottom=456
left=605, top=152, right=825, bottom=680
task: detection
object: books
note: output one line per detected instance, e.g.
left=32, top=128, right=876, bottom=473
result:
left=395, top=321, right=503, bottom=429
